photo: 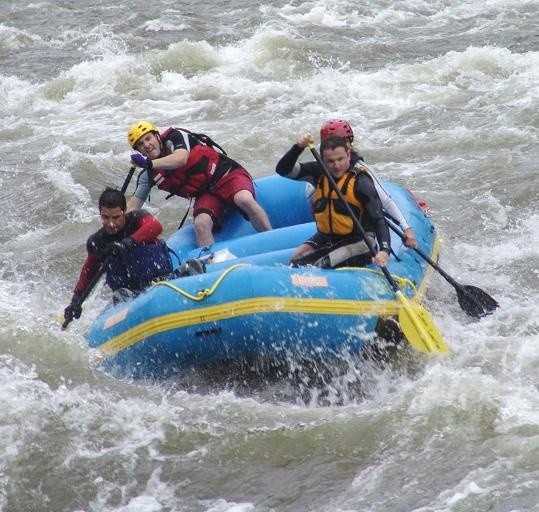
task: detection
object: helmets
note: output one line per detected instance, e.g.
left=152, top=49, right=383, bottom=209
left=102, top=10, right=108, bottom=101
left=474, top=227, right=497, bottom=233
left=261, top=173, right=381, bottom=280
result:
left=128, top=122, right=159, bottom=150
left=320, top=119, right=353, bottom=145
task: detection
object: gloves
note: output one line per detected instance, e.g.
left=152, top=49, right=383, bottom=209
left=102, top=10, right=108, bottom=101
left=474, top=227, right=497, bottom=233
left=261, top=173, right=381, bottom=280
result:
left=112, top=242, right=128, bottom=256
left=65, top=306, right=82, bottom=319
left=131, top=152, right=152, bottom=169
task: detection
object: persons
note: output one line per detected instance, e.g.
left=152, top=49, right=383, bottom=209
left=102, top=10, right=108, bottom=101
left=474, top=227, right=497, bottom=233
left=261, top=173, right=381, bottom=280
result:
left=62, top=188, right=175, bottom=324
left=118, top=118, right=274, bottom=250
left=315, top=117, right=421, bottom=251
left=276, top=131, right=393, bottom=272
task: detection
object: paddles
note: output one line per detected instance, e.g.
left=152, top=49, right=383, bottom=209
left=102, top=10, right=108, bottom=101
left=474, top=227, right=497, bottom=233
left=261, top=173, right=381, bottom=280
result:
left=308, top=143, right=448, bottom=353
left=385, top=215, right=497, bottom=320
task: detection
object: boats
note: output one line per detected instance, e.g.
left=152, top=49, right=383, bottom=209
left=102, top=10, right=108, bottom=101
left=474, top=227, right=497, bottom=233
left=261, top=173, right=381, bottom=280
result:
left=83, top=172, right=444, bottom=395
